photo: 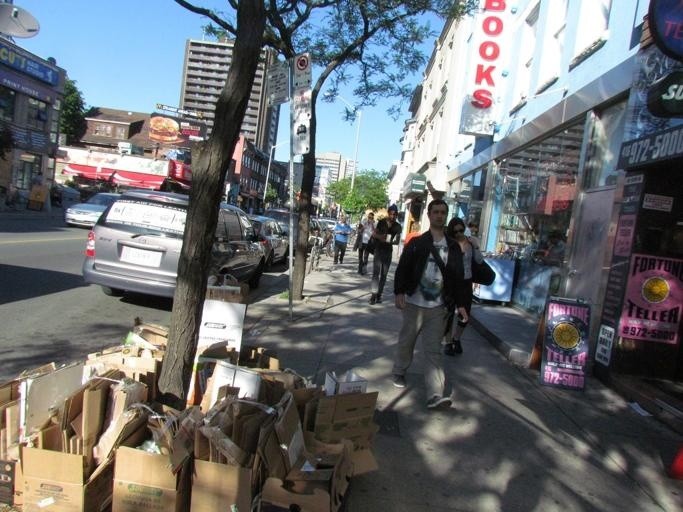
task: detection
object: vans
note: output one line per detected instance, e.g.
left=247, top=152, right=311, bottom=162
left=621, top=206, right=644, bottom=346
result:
left=83, top=191, right=267, bottom=295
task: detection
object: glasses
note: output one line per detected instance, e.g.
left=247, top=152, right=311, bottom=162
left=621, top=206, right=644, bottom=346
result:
left=453, top=229, right=464, bottom=233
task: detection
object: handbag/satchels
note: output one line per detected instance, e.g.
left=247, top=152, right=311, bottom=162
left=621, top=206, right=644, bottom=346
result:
left=446, top=269, right=463, bottom=300
left=472, top=260, right=496, bottom=285
left=367, top=237, right=376, bottom=254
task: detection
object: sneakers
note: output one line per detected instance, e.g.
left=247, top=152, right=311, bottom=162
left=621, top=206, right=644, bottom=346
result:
left=427, top=396, right=451, bottom=409
left=392, top=374, right=406, bottom=387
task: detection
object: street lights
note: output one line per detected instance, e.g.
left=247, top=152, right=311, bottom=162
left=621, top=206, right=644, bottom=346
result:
left=261, top=140, right=289, bottom=203
left=324, top=91, right=361, bottom=192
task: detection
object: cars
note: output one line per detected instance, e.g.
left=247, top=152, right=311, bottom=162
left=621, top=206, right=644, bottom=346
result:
left=262, top=209, right=338, bottom=251
left=248, top=216, right=290, bottom=271
left=63, top=191, right=122, bottom=231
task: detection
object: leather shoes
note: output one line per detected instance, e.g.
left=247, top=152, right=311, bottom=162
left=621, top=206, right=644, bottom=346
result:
left=452, top=338, right=462, bottom=354
left=445, top=343, right=454, bottom=355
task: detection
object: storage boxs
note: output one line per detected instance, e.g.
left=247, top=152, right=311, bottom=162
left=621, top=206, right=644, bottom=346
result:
left=186, top=299, right=247, bottom=408
left=0, top=415, right=264, bottom=511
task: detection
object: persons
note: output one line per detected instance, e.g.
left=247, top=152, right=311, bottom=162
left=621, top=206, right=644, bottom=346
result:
left=393, top=200, right=470, bottom=411
left=335, top=210, right=566, bottom=358
